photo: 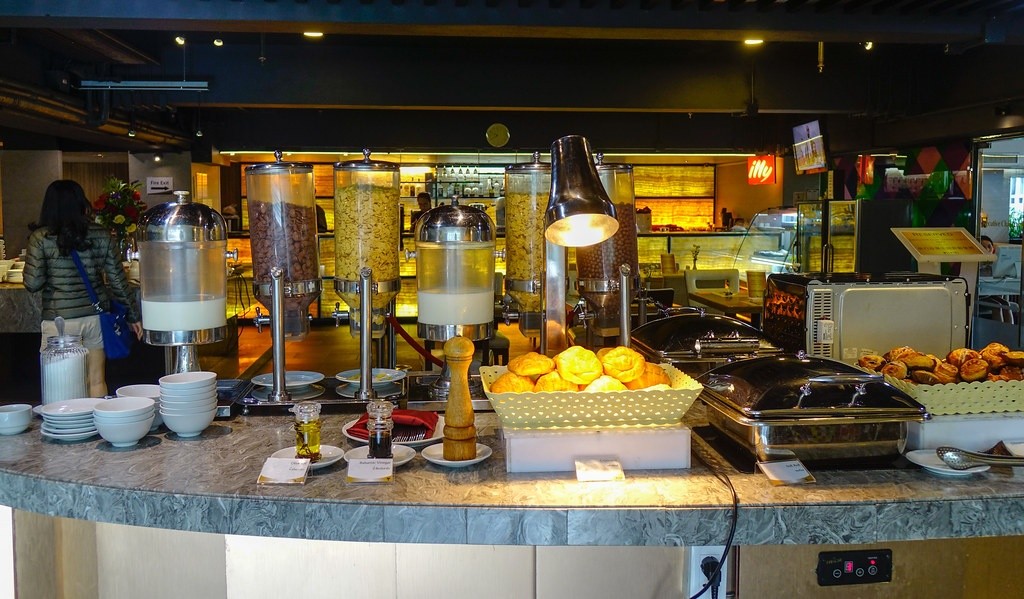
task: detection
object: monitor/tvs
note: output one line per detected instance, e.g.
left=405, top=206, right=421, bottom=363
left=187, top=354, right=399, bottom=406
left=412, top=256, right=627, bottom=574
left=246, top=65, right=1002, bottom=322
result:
left=792, top=119, right=831, bottom=177
left=992, top=245, right=1022, bottom=277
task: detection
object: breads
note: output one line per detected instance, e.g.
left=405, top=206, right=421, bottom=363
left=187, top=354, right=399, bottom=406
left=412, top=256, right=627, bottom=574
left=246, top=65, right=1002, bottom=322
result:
left=488, top=345, right=671, bottom=392
left=855, top=343, right=1024, bottom=385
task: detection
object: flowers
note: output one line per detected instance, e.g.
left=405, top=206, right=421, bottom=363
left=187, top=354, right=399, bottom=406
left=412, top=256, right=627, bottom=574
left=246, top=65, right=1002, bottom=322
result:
left=92, top=177, right=149, bottom=234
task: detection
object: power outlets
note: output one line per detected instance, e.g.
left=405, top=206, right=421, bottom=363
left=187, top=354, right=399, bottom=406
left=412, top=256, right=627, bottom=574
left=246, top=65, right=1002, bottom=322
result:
left=682, top=546, right=727, bottom=599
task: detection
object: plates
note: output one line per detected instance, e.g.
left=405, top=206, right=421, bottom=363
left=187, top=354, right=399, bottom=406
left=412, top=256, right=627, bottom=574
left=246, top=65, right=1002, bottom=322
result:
left=271, top=444, right=344, bottom=469
left=420, top=441, right=492, bottom=467
left=335, top=368, right=406, bottom=386
left=905, top=449, right=991, bottom=476
left=32, top=398, right=107, bottom=441
left=250, top=370, right=324, bottom=390
left=341, top=415, right=445, bottom=447
left=251, top=384, right=325, bottom=402
left=344, top=444, right=417, bottom=467
left=336, top=381, right=406, bottom=398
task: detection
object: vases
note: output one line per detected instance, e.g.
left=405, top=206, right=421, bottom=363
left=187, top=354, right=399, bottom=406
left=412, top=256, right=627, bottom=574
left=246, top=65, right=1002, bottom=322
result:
left=115, top=230, right=133, bottom=262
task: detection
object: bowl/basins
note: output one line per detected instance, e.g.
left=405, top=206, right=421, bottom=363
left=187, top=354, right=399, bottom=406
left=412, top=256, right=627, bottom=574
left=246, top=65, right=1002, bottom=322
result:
left=0, top=404, right=32, bottom=435
left=92, top=371, right=218, bottom=447
left=0, top=260, right=25, bottom=283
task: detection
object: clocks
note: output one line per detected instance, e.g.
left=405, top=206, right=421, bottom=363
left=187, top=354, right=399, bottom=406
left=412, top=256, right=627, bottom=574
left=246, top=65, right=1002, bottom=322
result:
left=486, top=123, right=511, bottom=148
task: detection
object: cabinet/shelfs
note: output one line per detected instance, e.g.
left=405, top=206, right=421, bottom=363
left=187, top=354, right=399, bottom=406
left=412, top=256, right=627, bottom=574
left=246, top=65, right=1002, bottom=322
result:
left=732, top=206, right=798, bottom=291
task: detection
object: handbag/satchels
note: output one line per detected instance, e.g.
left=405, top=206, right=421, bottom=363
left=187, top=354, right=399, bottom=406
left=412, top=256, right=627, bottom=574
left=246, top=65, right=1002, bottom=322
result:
left=99, top=302, right=134, bottom=359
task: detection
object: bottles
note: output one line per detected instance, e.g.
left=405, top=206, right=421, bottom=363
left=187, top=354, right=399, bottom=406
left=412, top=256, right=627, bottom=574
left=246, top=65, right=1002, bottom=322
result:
left=435, top=166, right=501, bottom=199
left=366, top=400, right=394, bottom=459
left=41, top=335, right=89, bottom=406
left=291, top=401, right=322, bottom=464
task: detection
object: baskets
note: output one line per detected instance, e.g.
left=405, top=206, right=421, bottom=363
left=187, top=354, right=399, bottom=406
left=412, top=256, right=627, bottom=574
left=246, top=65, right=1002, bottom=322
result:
left=853, top=357, right=1024, bottom=416
left=478, top=363, right=704, bottom=426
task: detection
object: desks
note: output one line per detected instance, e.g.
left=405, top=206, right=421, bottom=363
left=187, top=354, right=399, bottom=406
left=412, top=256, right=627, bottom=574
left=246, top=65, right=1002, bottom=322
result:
left=978, top=276, right=1021, bottom=326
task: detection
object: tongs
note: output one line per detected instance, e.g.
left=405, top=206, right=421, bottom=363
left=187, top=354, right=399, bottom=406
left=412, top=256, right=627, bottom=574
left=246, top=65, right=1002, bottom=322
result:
left=936, top=446, right=1024, bottom=470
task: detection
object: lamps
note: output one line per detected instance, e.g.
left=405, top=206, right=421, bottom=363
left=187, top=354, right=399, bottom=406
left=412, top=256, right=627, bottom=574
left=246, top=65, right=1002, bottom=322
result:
left=856, top=40, right=873, bottom=50
left=543, top=134, right=620, bottom=247
left=77, top=30, right=209, bottom=92
left=213, top=32, right=224, bottom=47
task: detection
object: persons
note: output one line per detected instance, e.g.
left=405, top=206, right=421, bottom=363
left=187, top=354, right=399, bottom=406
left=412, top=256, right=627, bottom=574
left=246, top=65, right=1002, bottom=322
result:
left=313, top=189, right=328, bottom=256
left=23, top=179, right=144, bottom=401
left=980, top=235, right=1014, bottom=325
left=408, top=190, right=431, bottom=233
